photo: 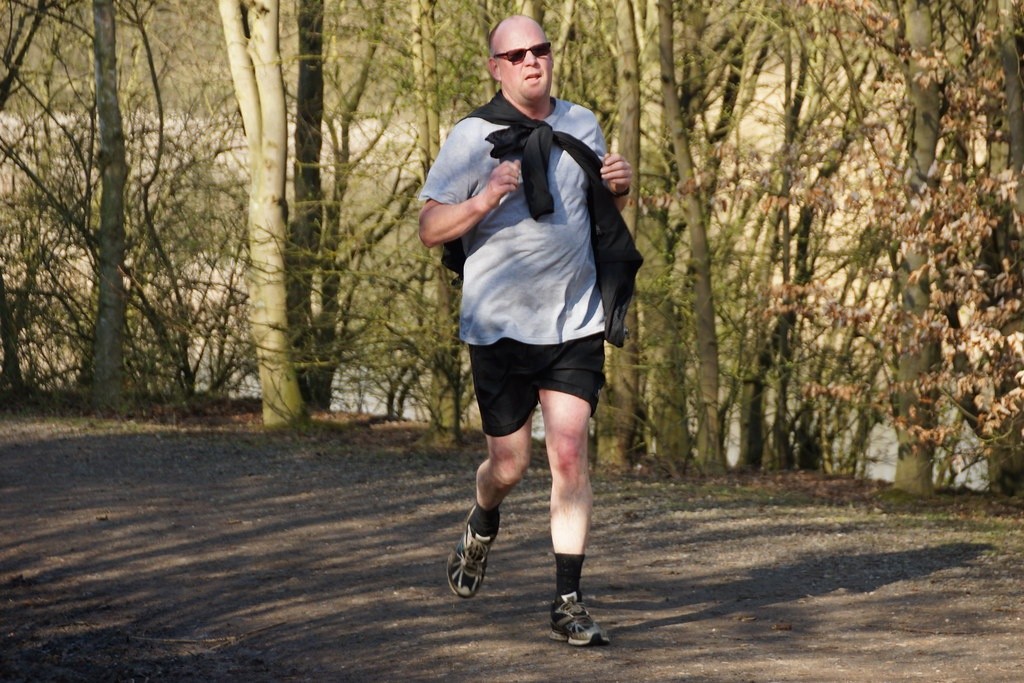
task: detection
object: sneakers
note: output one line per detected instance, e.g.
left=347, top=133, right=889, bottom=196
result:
left=547, top=592, right=610, bottom=647
left=447, top=504, right=500, bottom=598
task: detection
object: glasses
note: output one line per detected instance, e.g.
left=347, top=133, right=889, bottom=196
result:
left=492, top=41, right=551, bottom=64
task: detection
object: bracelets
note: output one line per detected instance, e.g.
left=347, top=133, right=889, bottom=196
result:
left=613, top=189, right=629, bottom=198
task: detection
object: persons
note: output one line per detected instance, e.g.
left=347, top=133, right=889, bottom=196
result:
left=418, top=15, right=644, bottom=645
